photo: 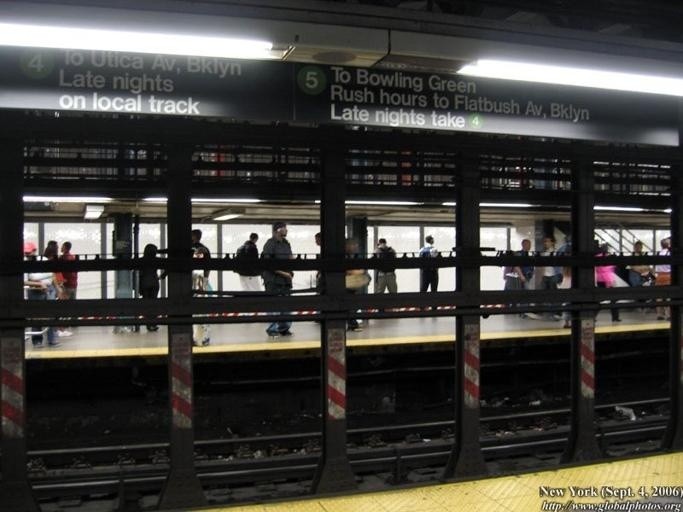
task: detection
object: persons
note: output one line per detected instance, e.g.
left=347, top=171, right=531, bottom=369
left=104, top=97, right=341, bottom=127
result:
left=373, top=238, right=397, bottom=293
left=499, top=236, right=671, bottom=321
left=314, top=232, right=325, bottom=292
left=136, top=243, right=168, bottom=331
left=42, top=239, right=64, bottom=298
left=418, top=236, right=443, bottom=311
left=190, top=229, right=211, bottom=347
left=157, top=247, right=170, bottom=281
left=258, top=221, right=294, bottom=339
left=372, top=243, right=381, bottom=294
left=341, top=237, right=364, bottom=332
left=59, top=241, right=78, bottom=301
left=234, top=232, right=264, bottom=292
left=22, top=243, right=67, bottom=349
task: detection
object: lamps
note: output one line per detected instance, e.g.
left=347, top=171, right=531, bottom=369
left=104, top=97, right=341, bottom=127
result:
left=361, top=9, right=683, bottom=104
left=0, top=0, right=393, bottom=101
